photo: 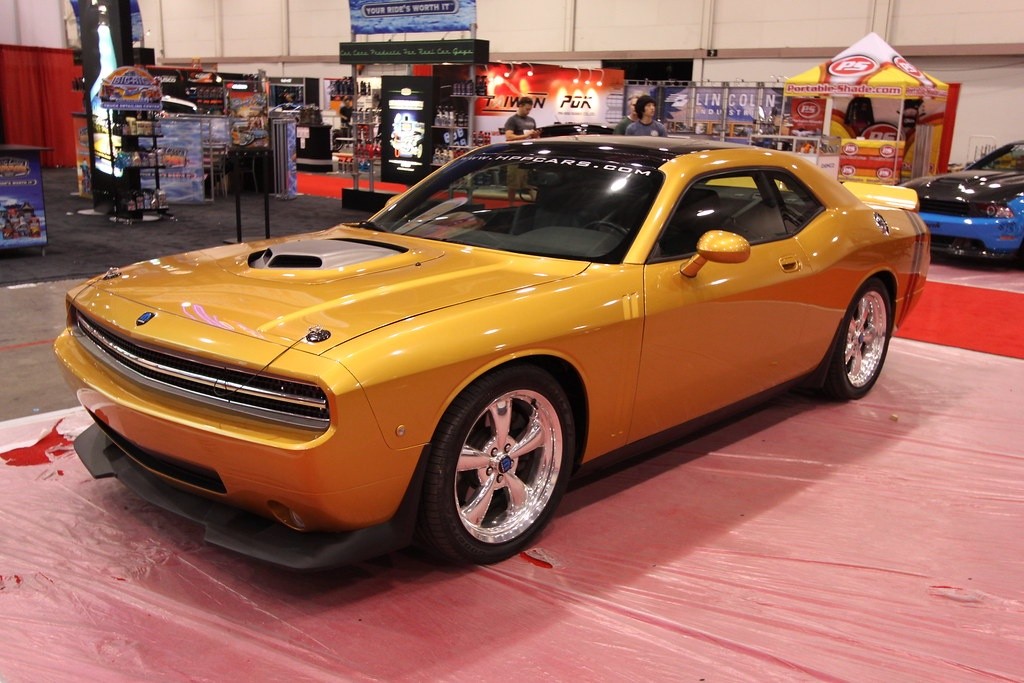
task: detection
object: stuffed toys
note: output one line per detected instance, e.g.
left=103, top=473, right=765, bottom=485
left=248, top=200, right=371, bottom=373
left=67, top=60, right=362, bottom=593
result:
left=896, top=99, right=926, bottom=127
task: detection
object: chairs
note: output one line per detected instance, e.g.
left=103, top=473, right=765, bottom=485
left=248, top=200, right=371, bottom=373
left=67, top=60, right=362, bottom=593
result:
left=507, top=169, right=618, bottom=238
left=203, top=143, right=227, bottom=199
left=665, top=190, right=724, bottom=256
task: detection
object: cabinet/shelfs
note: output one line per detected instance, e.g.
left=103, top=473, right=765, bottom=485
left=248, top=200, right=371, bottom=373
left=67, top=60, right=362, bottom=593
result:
left=107, top=108, right=174, bottom=224
left=430, top=63, right=496, bottom=204
left=331, top=67, right=381, bottom=193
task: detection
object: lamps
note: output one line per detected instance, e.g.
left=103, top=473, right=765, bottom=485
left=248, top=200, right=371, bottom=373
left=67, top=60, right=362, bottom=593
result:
left=585, top=69, right=592, bottom=85
left=504, top=63, right=514, bottom=77
left=596, top=70, right=604, bottom=86
left=573, top=68, right=581, bottom=84
left=527, top=63, right=534, bottom=77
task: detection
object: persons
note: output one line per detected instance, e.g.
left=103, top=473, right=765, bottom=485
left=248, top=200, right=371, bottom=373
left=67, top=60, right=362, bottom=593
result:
left=339, top=97, right=352, bottom=126
left=510, top=169, right=599, bottom=234
left=504, top=97, right=541, bottom=141
left=613, top=95, right=667, bottom=137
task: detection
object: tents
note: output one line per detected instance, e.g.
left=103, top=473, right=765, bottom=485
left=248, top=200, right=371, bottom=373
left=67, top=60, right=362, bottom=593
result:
left=778, top=32, right=950, bottom=188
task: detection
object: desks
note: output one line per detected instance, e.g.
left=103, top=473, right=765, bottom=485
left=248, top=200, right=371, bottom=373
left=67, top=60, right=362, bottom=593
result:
left=0, top=144, right=54, bottom=256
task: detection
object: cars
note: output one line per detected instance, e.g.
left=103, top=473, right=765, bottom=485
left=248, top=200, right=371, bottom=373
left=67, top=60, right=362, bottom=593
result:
left=536, top=122, right=616, bottom=138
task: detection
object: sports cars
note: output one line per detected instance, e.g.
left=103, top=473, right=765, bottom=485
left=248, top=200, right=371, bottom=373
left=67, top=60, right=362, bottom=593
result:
left=51, top=135, right=932, bottom=566
left=894, top=138, right=1024, bottom=269
